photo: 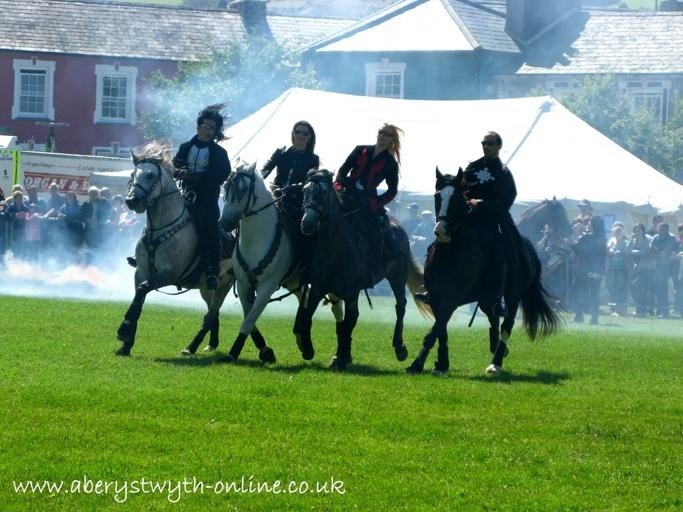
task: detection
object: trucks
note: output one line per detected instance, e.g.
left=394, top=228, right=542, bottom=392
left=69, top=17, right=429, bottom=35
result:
left=0, top=132, right=140, bottom=203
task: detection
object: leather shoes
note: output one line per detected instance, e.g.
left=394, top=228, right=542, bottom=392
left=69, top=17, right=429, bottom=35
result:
left=128, top=257, right=137, bottom=267
left=493, top=295, right=507, bottom=317
left=415, top=291, right=431, bottom=305
left=206, top=269, right=219, bottom=290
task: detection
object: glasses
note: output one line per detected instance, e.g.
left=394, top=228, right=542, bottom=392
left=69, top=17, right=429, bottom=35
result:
left=200, top=124, right=217, bottom=131
left=378, top=129, right=393, bottom=137
left=481, top=141, right=498, bottom=146
left=294, top=130, right=311, bottom=138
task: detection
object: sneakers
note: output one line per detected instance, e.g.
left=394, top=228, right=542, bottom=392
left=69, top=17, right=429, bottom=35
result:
left=609, top=309, right=681, bottom=320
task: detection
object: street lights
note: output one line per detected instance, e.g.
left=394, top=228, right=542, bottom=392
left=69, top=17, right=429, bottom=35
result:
left=33, top=119, right=72, bottom=151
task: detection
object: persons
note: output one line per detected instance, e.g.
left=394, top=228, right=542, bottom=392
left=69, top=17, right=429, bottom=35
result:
left=0, top=178, right=683, bottom=331
left=412, top=129, right=519, bottom=318
left=334, top=120, right=403, bottom=265
left=258, top=119, right=321, bottom=227
left=125, top=101, right=232, bottom=290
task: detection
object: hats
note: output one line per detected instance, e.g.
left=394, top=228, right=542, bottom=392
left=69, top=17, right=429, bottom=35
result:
left=406, top=203, right=418, bottom=210
left=577, top=199, right=593, bottom=211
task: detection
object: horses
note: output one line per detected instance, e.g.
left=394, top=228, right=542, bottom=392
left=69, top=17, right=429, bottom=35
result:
left=513, top=197, right=575, bottom=249
left=216, top=154, right=344, bottom=363
left=114, top=140, right=238, bottom=357
left=291, top=166, right=438, bottom=369
left=405, top=166, right=563, bottom=376
